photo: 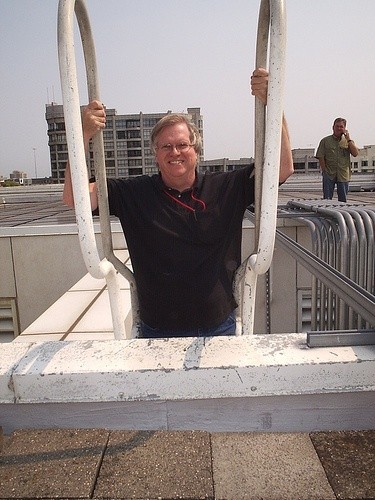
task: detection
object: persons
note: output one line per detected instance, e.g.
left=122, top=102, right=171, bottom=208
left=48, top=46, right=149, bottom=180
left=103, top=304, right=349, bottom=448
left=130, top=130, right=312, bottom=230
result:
left=63, top=68, right=295, bottom=339
left=316, top=117, right=358, bottom=202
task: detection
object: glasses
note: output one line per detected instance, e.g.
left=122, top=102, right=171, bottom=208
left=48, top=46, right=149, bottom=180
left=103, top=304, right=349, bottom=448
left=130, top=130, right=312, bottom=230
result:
left=155, top=142, right=195, bottom=154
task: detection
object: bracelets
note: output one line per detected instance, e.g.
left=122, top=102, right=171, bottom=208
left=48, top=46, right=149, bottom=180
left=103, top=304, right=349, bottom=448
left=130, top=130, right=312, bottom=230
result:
left=347, top=140, right=353, bottom=142
